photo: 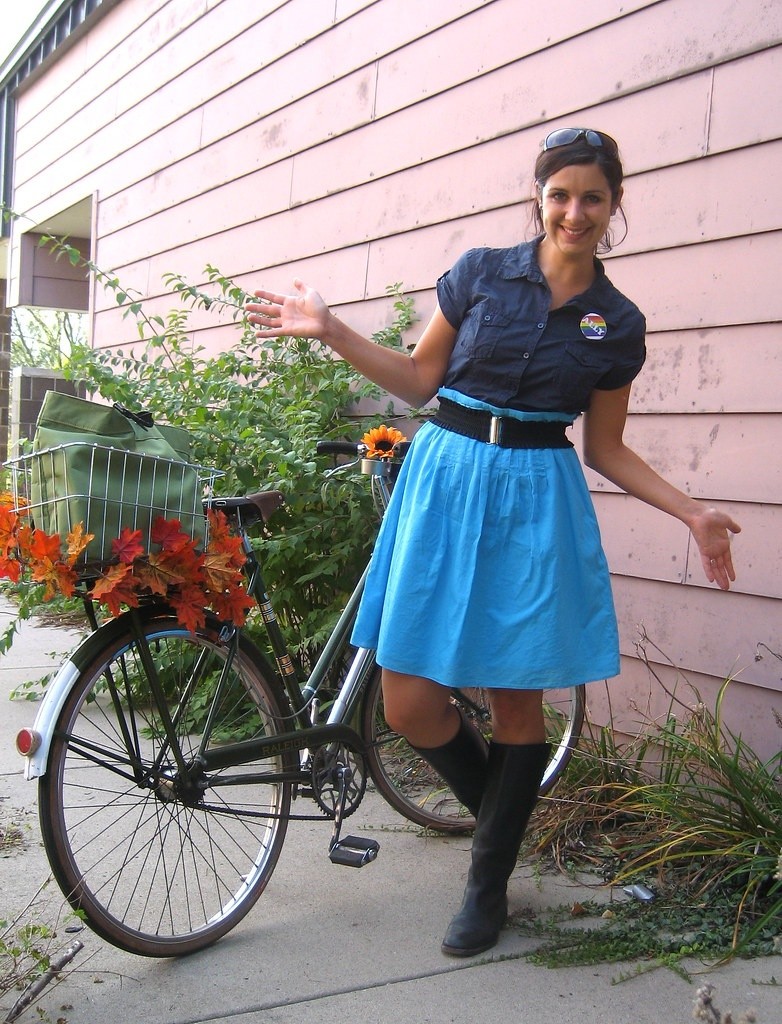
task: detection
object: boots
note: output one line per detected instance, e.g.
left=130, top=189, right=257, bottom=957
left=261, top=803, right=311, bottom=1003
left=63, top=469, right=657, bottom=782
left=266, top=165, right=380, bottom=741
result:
left=440, top=742, right=552, bottom=956
left=406, top=704, right=489, bottom=821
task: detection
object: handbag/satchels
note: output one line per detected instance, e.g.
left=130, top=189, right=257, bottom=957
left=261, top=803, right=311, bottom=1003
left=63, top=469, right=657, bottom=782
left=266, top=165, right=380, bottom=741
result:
left=29, top=386, right=206, bottom=563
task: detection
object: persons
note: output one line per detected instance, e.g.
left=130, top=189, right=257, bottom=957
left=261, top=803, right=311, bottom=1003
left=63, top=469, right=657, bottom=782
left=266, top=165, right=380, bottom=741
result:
left=245, top=126, right=744, bottom=956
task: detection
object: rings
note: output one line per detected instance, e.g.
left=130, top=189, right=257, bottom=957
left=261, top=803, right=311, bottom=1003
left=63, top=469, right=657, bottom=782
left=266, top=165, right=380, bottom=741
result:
left=709, top=558, right=717, bottom=563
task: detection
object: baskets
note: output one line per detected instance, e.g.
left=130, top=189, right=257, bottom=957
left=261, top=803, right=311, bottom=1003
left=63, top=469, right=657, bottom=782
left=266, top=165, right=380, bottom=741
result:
left=2, top=440, right=228, bottom=586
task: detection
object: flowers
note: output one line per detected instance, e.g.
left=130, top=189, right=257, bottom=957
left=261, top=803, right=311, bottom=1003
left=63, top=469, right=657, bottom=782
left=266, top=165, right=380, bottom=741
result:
left=362, top=425, right=408, bottom=460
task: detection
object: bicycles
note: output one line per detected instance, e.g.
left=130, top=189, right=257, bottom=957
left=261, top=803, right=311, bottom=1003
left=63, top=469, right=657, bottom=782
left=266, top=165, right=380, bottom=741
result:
left=2, top=438, right=590, bottom=960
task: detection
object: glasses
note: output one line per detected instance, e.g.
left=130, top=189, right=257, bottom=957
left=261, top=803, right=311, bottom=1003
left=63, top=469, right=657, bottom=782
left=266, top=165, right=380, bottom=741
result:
left=543, top=127, right=620, bottom=159
